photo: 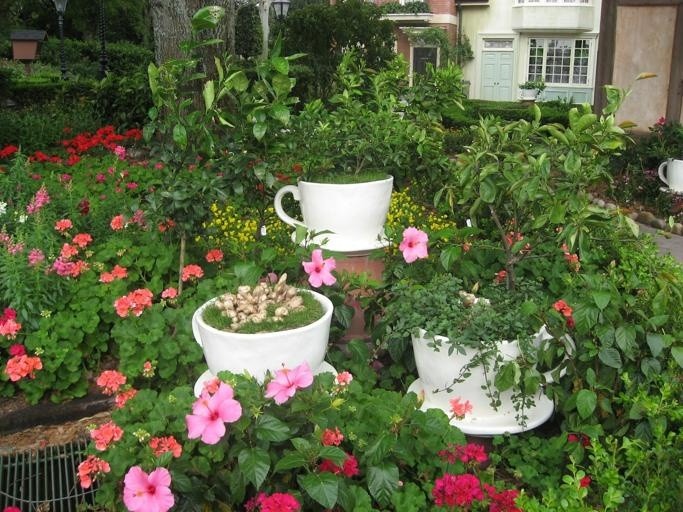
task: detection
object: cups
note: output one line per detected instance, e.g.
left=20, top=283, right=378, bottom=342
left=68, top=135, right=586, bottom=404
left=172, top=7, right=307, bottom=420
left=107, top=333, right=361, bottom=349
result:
left=273, top=169, right=395, bottom=245
left=410, top=313, right=577, bottom=420
left=192, top=286, right=335, bottom=384
left=659, top=158, right=683, bottom=191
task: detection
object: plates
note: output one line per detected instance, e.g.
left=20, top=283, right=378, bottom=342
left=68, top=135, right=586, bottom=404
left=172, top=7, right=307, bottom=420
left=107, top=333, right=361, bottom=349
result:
left=659, top=187, right=683, bottom=196
left=406, top=375, right=555, bottom=437
left=194, top=362, right=340, bottom=400
left=291, top=229, right=394, bottom=253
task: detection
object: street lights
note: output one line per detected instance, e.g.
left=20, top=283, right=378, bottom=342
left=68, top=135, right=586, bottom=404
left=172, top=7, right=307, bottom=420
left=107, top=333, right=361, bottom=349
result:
left=51, top=0, right=70, bottom=80
left=272, top=0, right=290, bottom=57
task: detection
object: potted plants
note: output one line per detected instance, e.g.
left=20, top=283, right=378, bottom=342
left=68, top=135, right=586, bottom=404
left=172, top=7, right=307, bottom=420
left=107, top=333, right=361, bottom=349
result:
left=640, top=115, right=683, bottom=196
left=363, top=274, right=578, bottom=440
left=187, top=272, right=340, bottom=397
left=261, top=89, right=448, bottom=253
